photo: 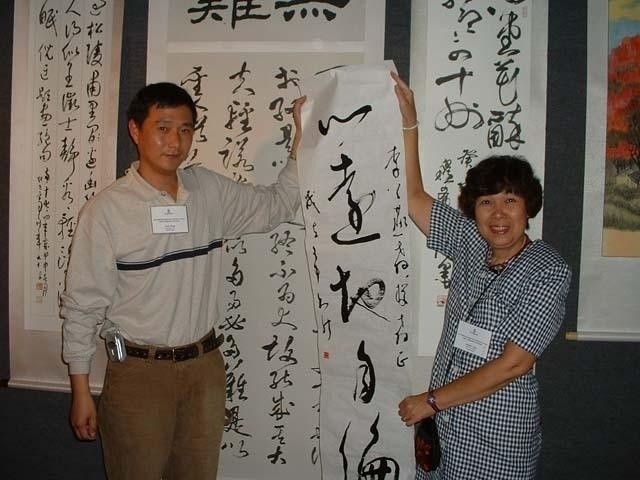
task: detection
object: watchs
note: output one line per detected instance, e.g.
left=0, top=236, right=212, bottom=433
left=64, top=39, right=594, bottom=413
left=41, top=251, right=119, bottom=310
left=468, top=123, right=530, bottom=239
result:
left=427, top=389, right=441, bottom=413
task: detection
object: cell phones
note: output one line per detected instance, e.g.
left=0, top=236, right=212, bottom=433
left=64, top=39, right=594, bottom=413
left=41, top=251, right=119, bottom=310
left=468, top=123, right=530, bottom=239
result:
left=102, top=328, right=125, bottom=361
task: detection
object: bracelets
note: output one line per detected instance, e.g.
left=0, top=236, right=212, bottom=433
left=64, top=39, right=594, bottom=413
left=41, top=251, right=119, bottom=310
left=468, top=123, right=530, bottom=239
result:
left=401, top=120, right=420, bottom=132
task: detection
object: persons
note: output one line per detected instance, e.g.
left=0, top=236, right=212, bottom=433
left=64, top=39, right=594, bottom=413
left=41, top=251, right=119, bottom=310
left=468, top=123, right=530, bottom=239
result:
left=391, top=71, right=573, bottom=480
left=60, top=84, right=307, bottom=480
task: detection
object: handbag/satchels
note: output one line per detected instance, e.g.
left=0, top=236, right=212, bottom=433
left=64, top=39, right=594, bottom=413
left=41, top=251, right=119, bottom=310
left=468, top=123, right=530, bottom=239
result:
left=415, top=413, right=442, bottom=473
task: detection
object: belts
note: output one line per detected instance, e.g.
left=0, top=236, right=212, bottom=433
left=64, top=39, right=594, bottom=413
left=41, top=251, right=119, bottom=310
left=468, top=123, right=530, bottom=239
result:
left=126, top=328, right=224, bottom=362
